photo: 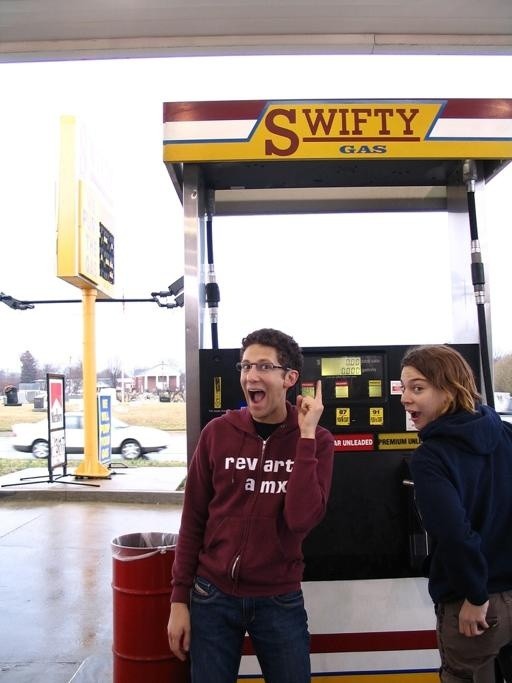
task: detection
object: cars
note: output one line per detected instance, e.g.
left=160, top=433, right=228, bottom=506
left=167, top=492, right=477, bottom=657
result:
left=11, top=412, right=168, bottom=459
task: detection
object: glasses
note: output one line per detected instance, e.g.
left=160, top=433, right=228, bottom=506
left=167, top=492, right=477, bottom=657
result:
left=234, top=360, right=293, bottom=373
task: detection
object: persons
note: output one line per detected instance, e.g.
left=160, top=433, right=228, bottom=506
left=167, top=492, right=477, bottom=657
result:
left=397, top=344, right=512, bottom=683
left=167, top=326, right=334, bottom=683
